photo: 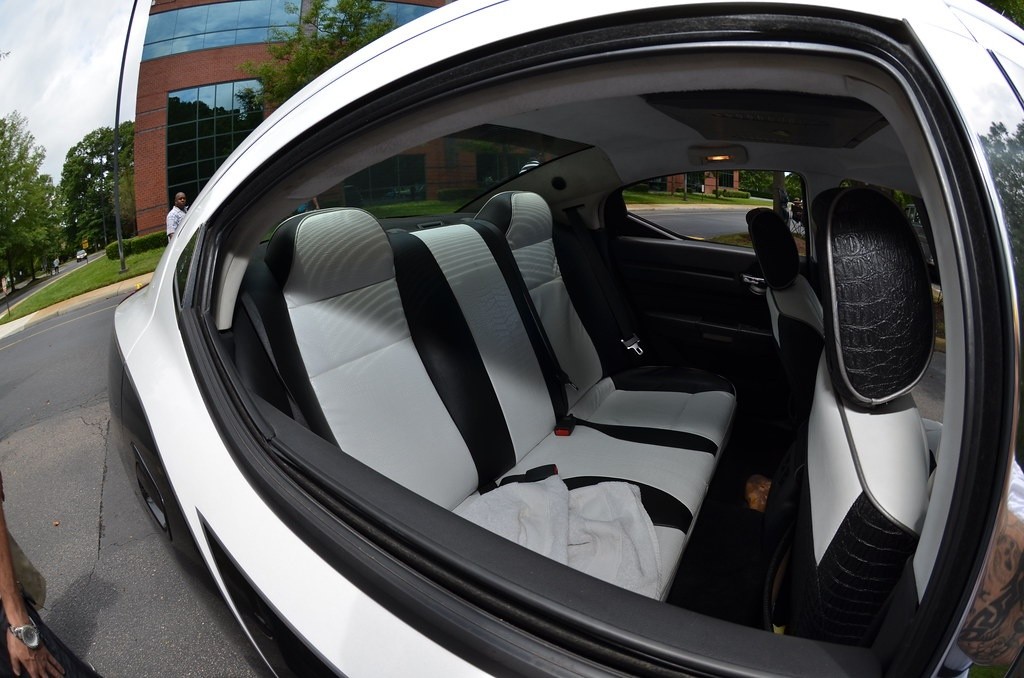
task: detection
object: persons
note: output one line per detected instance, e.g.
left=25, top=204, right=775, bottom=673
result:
left=0, top=479, right=106, bottom=678
left=295, top=196, right=322, bottom=216
left=164, top=190, right=188, bottom=244
left=53, top=256, right=60, bottom=274
left=791, top=197, right=804, bottom=223
left=519, top=151, right=544, bottom=177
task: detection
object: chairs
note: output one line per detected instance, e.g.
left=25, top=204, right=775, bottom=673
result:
left=746, top=205, right=826, bottom=457
left=766, top=184, right=936, bottom=651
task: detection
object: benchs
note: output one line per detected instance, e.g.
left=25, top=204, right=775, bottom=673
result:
left=257, top=187, right=739, bottom=608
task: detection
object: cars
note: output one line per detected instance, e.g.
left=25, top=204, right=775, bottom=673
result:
left=76, top=250, right=88, bottom=262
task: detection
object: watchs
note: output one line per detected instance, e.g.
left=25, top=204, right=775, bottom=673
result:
left=6, top=617, right=42, bottom=649
